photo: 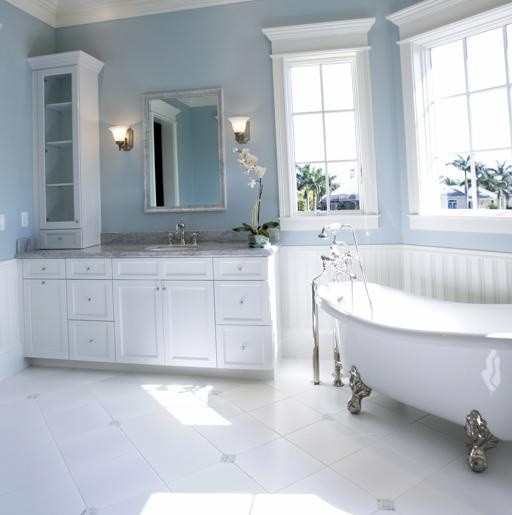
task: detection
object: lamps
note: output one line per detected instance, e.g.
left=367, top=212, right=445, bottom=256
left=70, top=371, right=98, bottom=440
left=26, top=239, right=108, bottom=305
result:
left=228, top=111, right=251, bottom=144
left=106, top=124, right=136, bottom=153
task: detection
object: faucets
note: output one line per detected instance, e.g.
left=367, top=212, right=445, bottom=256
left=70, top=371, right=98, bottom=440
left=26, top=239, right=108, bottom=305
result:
left=176, top=224, right=185, bottom=240
left=335, top=267, right=356, bottom=279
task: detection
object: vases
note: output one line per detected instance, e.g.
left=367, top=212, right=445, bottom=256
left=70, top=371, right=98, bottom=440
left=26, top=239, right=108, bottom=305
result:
left=248, top=233, right=270, bottom=248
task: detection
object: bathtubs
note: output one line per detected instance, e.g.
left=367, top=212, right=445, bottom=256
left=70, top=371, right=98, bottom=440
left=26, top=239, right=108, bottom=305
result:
left=314, top=280, right=512, bottom=473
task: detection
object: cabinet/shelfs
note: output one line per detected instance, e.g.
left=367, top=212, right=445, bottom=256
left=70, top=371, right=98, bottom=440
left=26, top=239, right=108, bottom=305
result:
left=114, top=259, right=214, bottom=367
left=215, top=258, right=279, bottom=371
left=26, top=49, right=107, bottom=251
left=22, top=259, right=70, bottom=362
left=69, top=257, right=115, bottom=363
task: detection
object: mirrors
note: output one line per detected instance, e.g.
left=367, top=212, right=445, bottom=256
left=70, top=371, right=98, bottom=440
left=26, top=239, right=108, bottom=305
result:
left=138, top=86, right=231, bottom=215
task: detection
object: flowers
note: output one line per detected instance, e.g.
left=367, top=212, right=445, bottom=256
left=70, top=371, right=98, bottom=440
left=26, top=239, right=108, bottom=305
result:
left=229, top=148, right=282, bottom=241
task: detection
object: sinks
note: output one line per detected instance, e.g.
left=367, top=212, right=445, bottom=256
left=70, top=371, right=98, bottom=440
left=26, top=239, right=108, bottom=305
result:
left=145, top=245, right=198, bottom=250
